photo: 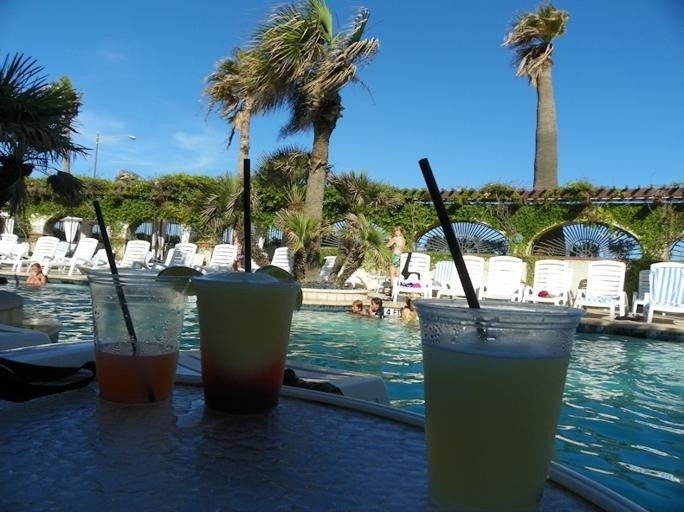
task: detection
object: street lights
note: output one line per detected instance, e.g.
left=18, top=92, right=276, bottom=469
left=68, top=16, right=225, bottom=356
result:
left=92, top=133, right=137, bottom=178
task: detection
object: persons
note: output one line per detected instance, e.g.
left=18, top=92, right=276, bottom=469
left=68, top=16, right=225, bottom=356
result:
left=386, top=223, right=404, bottom=298
left=26, top=263, right=47, bottom=285
left=351, top=293, right=419, bottom=323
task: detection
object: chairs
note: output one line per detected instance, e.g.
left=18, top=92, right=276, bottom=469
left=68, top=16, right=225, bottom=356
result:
left=319, top=251, right=683, bottom=326
left=0, top=234, right=295, bottom=277
left=2, top=325, right=391, bottom=406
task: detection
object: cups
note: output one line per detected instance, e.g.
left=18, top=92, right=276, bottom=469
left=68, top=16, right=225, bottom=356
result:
left=413, top=296, right=587, bottom=512
left=81, top=268, right=192, bottom=403
left=192, top=275, right=303, bottom=416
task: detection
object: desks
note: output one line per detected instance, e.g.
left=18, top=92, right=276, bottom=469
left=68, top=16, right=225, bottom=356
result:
left=0, top=371, right=649, bottom=512
